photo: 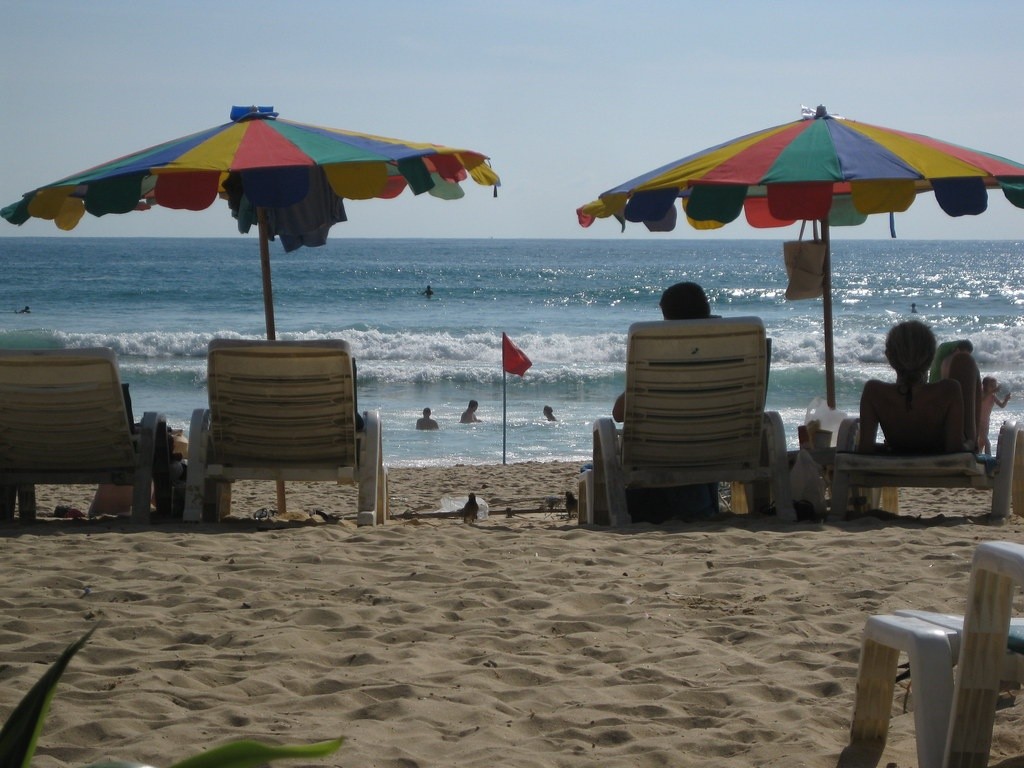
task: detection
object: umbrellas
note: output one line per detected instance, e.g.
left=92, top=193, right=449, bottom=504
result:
left=574, top=103, right=1024, bottom=412
left=0, top=102, right=502, bottom=515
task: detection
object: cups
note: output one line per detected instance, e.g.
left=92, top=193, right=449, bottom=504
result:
left=798, top=426, right=815, bottom=450
left=814, top=429, right=834, bottom=450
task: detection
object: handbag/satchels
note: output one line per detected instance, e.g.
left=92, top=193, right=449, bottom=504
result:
left=783, top=219, right=826, bottom=301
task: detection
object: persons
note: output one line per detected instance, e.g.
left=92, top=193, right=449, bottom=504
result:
left=543, top=405, right=556, bottom=421
left=422, top=285, right=434, bottom=296
left=978, top=376, right=1010, bottom=456
left=855, top=321, right=981, bottom=456
left=18, top=305, right=30, bottom=313
left=460, top=399, right=481, bottom=423
left=611, top=280, right=711, bottom=421
left=415, top=406, right=438, bottom=430
left=88, top=483, right=134, bottom=517
left=911, top=302, right=918, bottom=313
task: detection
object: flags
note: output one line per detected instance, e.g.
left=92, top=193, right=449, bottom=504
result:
left=502, top=333, right=533, bottom=377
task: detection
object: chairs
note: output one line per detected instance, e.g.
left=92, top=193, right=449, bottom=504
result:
left=833, top=408, right=1023, bottom=521
left=187, top=335, right=393, bottom=521
left=0, top=346, right=179, bottom=512
left=583, top=314, right=790, bottom=544
left=850, top=544, right=1024, bottom=768
